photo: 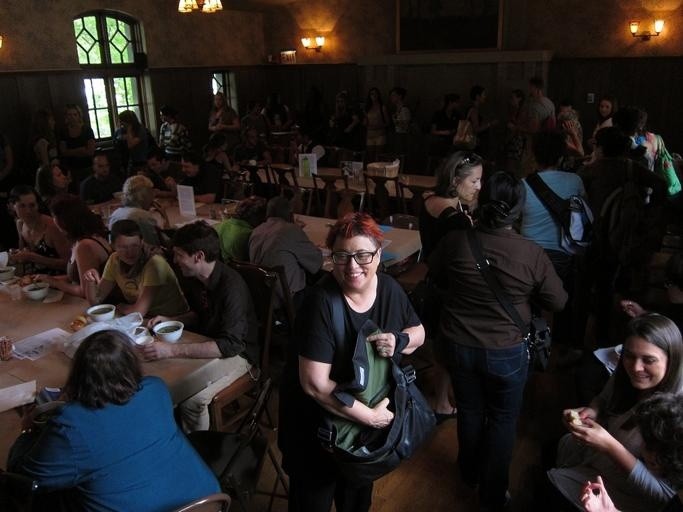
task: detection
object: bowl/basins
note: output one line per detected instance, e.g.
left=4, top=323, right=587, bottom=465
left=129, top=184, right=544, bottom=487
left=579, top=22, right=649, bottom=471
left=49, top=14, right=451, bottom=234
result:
left=86, top=302, right=116, bottom=323
left=0, top=265, right=17, bottom=282
left=21, top=282, right=50, bottom=302
left=112, top=192, right=123, bottom=200
left=152, top=320, right=184, bottom=343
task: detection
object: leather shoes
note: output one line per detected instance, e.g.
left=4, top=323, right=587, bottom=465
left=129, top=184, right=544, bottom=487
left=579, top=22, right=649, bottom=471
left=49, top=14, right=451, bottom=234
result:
left=434, top=406, right=457, bottom=421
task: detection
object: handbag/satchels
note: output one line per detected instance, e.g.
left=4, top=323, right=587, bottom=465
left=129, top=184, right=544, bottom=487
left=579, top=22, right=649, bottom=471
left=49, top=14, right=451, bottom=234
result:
left=21, top=388, right=64, bottom=431
left=527, top=318, right=553, bottom=369
left=560, top=194, right=596, bottom=257
left=317, top=320, right=436, bottom=480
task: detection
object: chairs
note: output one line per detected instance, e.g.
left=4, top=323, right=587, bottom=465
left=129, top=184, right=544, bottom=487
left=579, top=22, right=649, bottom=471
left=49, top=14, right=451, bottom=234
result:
left=0, top=128, right=491, bottom=512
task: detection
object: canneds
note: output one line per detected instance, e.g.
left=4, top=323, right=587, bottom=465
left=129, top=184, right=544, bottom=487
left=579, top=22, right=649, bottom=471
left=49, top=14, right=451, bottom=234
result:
left=0, top=336, right=16, bottom=361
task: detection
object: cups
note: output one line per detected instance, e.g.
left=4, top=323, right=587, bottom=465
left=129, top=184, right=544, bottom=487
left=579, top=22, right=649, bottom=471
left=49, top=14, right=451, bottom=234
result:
left=614, top=343, right=621, bottom=362
left=134, top=334, right=154, bottom=362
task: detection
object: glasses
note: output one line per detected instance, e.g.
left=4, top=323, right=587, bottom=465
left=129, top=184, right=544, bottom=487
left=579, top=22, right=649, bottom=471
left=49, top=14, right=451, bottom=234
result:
left=455, top=154, right=476, bottom=177
left=330, top=247, right=380, bottom=265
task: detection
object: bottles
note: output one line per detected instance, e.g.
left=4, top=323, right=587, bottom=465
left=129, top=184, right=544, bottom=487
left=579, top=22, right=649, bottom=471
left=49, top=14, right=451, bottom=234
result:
left=664, top=160, right=670, bottom=168
left=300, top=155, right=311, bottom=177
left=350, top=162, right=363, bottom=186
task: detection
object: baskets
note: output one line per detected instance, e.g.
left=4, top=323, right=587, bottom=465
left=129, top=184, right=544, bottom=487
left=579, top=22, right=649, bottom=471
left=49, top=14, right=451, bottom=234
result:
left=367, top=162, right=399, bottom=178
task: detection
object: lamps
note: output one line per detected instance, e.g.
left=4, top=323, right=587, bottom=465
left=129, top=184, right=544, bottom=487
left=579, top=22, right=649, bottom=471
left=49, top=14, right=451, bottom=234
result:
left=628, top=19, right=664, bottom=41
left=175, top=0, right=224, bottom=13
left=301, top=36, right=325, bottom=53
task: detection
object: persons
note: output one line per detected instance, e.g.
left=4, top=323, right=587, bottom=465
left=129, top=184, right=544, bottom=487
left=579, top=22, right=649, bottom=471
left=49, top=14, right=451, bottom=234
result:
left=6, top=330, right=222, bottom=512
left=277, top=212, right=426, bottom=511
left=2, top=102, right=321, bottom=321
left=540, top=313, right=682, bottom=512
left=158, top=85, right=415, bottom=180
left=418, top=150, right=483, bottom=425
left=579, top=393, right=682, bottom=512
left=430, top=77, right=680, bottom=359
left=134, top=221, right=259, bottom=435
left=426, top=173, right=567, bottom=511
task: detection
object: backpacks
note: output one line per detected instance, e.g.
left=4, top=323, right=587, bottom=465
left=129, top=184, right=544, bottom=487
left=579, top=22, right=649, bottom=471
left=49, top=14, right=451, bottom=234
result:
left=600, top=158, right=653, bottom=269
